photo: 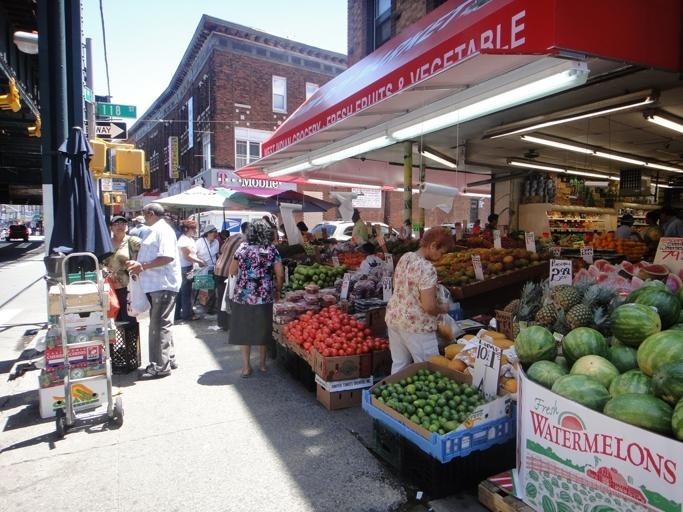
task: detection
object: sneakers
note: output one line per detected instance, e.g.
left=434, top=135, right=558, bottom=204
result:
left=139, top=369, right=171, bottom=380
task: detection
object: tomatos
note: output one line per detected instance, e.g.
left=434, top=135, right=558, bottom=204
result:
left=283, top=304, right=390, bottom=356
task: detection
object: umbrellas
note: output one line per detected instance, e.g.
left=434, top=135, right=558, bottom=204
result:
left=48, top=126, right=115, bottom=281
left=151, top=184, right=338, bottom=238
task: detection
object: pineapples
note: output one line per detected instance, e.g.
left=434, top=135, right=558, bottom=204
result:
left=503, top=274, right=625, bottom=343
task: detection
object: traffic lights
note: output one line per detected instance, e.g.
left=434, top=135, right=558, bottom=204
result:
left=0, top=92, right=21, bottom=112
left=27, top=122, right=41, bottom=138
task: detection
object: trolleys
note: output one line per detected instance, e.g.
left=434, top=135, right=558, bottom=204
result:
left=41, top=248, right=124, bottom=439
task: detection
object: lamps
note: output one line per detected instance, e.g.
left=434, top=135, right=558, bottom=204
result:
left=479, top=89, right=659, bottom=146
left=507, top=109, right=682, bottom=190
left=419, top=140, right=457, bottom=170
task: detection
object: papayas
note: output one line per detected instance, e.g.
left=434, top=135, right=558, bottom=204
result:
left=431, top=320, right=518, bottom=394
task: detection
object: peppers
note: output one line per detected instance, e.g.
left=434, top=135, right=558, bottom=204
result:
left=434, top=248, right=519, bottom=286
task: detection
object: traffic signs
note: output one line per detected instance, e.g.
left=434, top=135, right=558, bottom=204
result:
left=93, top=102, right=138, bottom=119
left=84, top=122, right=127, bottom=140
left=84, top=86, right=95, bottom=103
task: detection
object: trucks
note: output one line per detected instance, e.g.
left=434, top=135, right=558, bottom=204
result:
left=186, top=211, right=280, bottom=243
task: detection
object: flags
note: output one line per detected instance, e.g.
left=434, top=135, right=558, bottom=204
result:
left=265, top=189, right=339, bottom=213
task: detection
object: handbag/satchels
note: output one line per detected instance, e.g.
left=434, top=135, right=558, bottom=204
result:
left=194, top=274, right=216, bottom=289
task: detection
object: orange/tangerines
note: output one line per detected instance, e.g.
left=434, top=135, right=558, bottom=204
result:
left=371, top=368, right=492, bottom=436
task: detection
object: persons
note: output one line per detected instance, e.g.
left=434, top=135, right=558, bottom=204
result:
left=107, top=202, right=312, bottom=378
left=614, top=206, right=683, bottom=242
left=472, top=220, right=481, bottom=233
left=385, top=228, right=454, bottom=376
left=352, top=208, right=368, bottom=241
left=486, top=214, right=498, bottom=229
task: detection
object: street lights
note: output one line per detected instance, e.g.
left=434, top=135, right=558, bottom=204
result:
left=12, top=28, right=95, bottom=190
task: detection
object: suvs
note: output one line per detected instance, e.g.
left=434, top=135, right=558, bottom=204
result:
left=310, top=218, right=400, bottom=243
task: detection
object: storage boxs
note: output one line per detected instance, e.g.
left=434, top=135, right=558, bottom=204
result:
left=198, top=295, right=212, bottom=305
left=37, top=283, right=119, bottom=418
left=314, top=370, right=374, bottom=412
left=513, top=364, right=683, bottom=511
left=198, top=288, right=214, bottom=296
left=360, top=358, right=512, bottom=445
left=312, top=347, right=371, bottom=379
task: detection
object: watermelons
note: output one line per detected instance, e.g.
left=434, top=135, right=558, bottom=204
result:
left=526, top=469, right=637, bottom=512
left=515, top=259, right=683, bottom=447
left=646, top=226, right=663, bottom=242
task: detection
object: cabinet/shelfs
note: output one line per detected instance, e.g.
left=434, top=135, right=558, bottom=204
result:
left=517, top=203, right=617, bottom=242
left=616, top=202, right=663, bottom=224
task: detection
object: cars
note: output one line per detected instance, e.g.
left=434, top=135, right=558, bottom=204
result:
left=5, top=225, right=35, bottom=244
left=411, top=220, right=483, bottom=239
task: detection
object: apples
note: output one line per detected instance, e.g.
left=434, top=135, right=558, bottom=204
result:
left=290, top=263, right=346, bottom=291
left=488, top=249, right=548, bottom=276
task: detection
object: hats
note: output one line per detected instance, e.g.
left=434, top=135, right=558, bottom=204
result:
left=131, top=215, right=146, bottom=224
left=111, top=215, right=130, bottom=224
left=204, top=224, right=217, bottom=235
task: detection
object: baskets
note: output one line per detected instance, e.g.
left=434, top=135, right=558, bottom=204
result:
left=108, top=322, right=140, bottom=375
